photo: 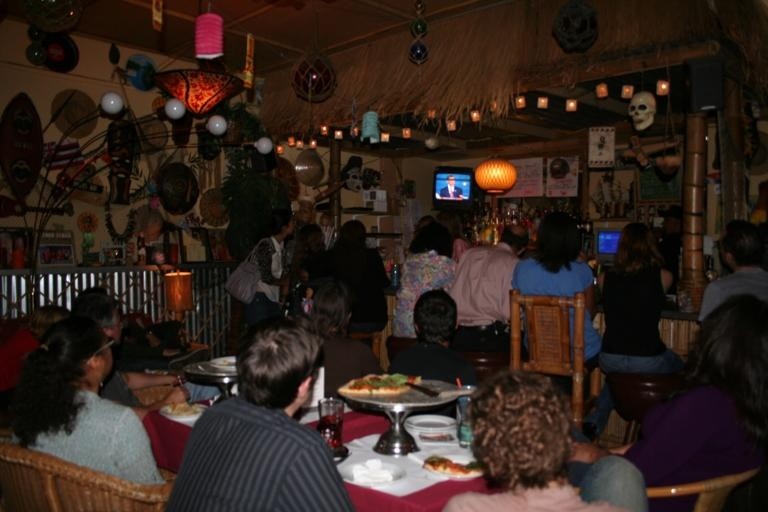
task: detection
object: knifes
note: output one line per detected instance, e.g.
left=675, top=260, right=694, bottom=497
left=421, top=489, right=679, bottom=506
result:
left=405, top=381, right=439, bottom=398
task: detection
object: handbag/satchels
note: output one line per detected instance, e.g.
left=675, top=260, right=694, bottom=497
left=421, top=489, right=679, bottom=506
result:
left=224, top=260, right=262, bottom=305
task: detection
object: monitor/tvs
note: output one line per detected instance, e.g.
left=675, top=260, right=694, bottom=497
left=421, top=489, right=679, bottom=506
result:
left=594, top=230, right=624, bottom=256
left=432, top=167, right=482, bottom=208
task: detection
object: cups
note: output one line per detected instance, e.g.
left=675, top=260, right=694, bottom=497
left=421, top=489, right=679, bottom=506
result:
left=318, top=397, right=343, bottom=452
left=456, top=384, right=476, bottom=448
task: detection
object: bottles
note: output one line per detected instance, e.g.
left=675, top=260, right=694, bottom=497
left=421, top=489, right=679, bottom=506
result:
left=594, top=262, right=605, bottom=298
left=599, top=199, right=626, bottom=218
left=471, top=206, right=593, bottom=247
left=391, top=261, right=400, bottom=286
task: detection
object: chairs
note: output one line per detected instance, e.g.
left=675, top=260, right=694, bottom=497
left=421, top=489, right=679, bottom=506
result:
left=0, top=286, right=768, bottom=512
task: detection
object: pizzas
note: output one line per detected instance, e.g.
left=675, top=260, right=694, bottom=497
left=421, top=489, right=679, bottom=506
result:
left=339, top=373, right=422, bottom=395
left=424, top=456, right=470, bottom=475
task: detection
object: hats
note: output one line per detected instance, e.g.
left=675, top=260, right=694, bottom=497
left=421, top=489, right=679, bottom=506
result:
left=135, top=115, right=169, bottom=155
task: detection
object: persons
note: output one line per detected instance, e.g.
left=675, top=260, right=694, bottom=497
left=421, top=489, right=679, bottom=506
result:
left=1, top=212, right=767, bottom=511
left=440, top=175, right=463, bottom=200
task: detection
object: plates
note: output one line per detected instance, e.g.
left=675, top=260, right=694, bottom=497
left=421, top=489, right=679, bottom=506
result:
left=270, top=145, right=328, bottom=224
left=157, top=403, right=210, bottom=421
left=141, top=119, right=168, bottom=155
left=41, top=31, right=81, bottom=73
left=126, top=53, right=157, bottom=92
left=209, top=355, right=237, bottom=370
left=406, top=413, right=457, bottom=432
left=405, top=448, right=484, bottom=479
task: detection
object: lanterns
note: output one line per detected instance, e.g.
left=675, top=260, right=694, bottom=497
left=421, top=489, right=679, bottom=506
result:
left=474, top=157, right=518, bottom=194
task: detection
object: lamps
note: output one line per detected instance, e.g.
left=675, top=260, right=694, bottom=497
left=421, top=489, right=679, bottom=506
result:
left=150, top=36, right=247, bottom=123
left=473, top=157, right=520, bottom=222
left=276, top=66, right=671, bottom=155
